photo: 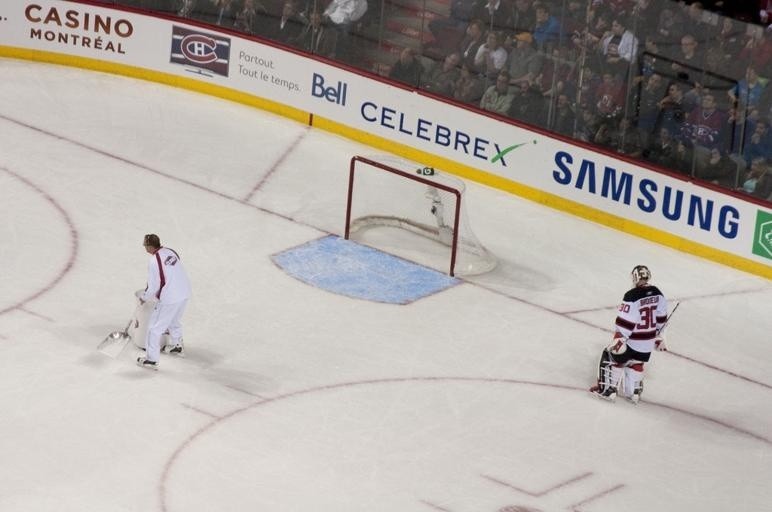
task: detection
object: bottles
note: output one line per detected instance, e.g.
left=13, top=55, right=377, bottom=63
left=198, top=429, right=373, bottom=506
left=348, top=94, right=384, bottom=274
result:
left=514, top=31, right=533, bottom=45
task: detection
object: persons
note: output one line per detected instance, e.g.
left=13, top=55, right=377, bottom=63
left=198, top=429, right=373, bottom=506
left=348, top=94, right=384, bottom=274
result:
left=390, top=2, right=771, bottom=202
left=169, top=2, right=201, bottom=20
left=136, top=234, right=191, bottom=366
left=203, top=2, right=234, bottom=28
left=322, top=1, right=368, bottom=58
left=590, top=265, right=668, bottom=405
left=233, top=2, right=271, bottom=38
left=266, top=2, right=303, bottom=43
left=295, top=7, right=338, bottom=60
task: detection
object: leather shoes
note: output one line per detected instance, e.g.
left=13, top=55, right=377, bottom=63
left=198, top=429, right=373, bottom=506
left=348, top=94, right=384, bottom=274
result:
left=607, top=332, right=628, bottom=355
left=654, top=336, right=667, bottom=351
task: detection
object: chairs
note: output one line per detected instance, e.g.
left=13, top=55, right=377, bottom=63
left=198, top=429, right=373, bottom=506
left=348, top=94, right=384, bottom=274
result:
left=498, top=0, right=771, bottom=204
left=175, top=0, right=498, bottom=116
left=162, top=344, right=182, bottom=354
left=137, top=357, right=156, bottom=365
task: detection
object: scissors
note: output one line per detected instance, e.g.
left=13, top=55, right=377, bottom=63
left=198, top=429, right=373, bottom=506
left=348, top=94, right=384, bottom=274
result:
left=97, top=303, right=140, bottom=361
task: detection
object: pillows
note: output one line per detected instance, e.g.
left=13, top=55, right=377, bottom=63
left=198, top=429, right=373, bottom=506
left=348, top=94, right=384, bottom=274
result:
left=631, top=265, right=651, bottom=285
